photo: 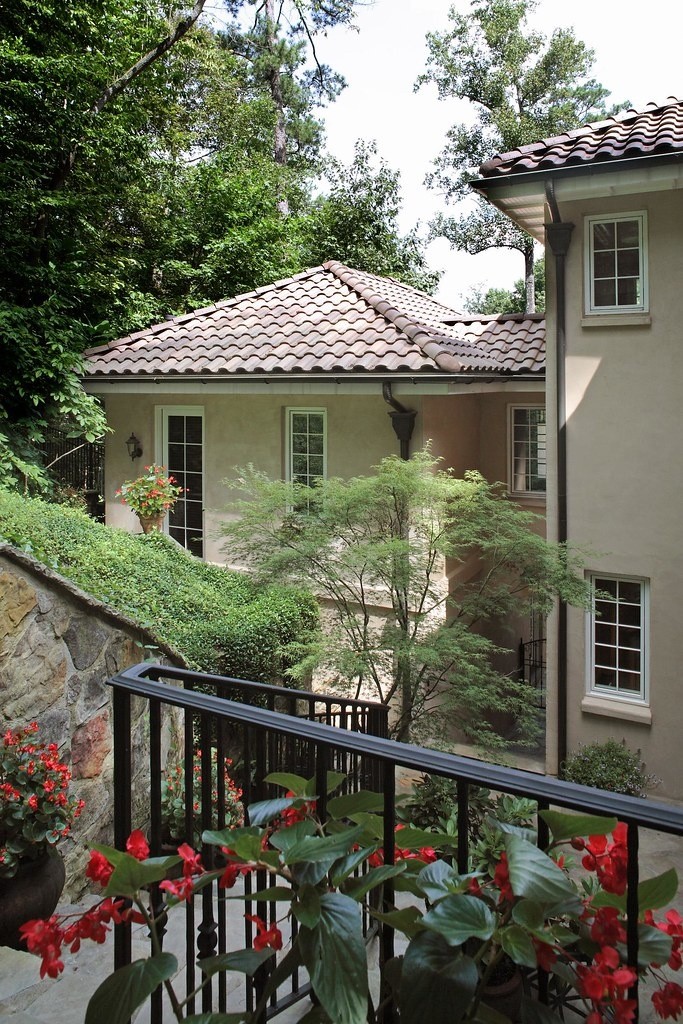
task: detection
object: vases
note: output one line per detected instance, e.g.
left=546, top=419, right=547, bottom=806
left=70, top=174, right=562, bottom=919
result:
left=475, top=967, right=524, bottom=1018
left=136, top=508, right=166, bottom=534
left=0, top=840, right=67, bottom=942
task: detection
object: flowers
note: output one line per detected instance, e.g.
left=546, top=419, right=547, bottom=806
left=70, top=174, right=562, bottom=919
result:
left=17, top=786, right=682, bottom=1024
left=114, top=463, right=189, bottom=516
left=0, top=721, right=87, bottom=879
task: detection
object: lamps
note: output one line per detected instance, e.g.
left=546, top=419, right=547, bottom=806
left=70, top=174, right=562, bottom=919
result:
left=126, top=432, right=144, bottom=461
